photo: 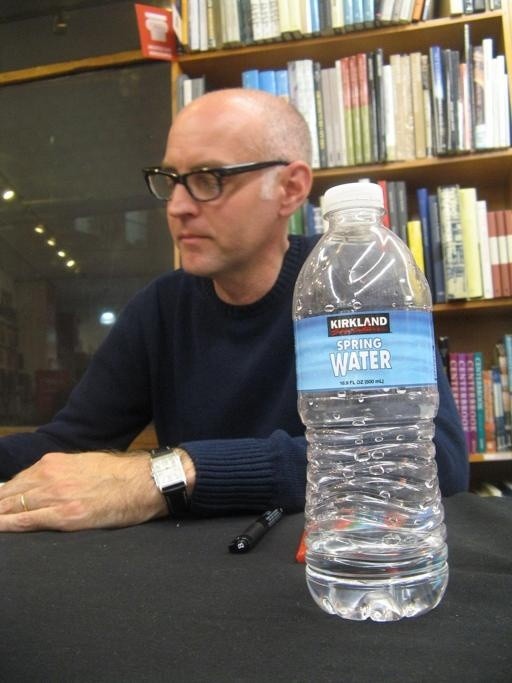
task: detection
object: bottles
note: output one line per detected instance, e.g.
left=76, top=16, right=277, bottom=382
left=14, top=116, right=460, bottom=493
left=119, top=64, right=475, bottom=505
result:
left=290, top=179, right=451, bottom=624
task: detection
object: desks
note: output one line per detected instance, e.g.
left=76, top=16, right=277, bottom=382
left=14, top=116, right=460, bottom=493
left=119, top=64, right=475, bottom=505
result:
left=1, top=492, right=512, bottom=683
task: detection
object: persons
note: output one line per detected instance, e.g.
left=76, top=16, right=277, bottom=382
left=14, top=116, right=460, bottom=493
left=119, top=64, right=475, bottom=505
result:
left=0, top=86, right=469, bottom=532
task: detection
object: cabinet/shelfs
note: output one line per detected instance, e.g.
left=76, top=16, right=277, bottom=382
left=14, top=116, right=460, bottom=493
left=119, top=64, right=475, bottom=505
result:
left=169, top=1, right=512, bottom=493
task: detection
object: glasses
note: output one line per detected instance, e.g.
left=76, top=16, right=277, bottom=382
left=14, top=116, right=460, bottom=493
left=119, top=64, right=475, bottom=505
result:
left=142, top=160, right=291, bottom=202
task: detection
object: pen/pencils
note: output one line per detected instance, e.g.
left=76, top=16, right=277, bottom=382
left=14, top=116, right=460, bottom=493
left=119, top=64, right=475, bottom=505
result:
left=295, top=531, right=305, bottom=564
left=228, top=506, right=284, bottom=553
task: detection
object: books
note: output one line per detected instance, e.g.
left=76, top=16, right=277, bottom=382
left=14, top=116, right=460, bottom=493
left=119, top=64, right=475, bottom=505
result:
left=288, top=176, right=512, bottom=304
left=175, top=2, right=502, bottom=56
left=175, top=24, right=510, bottom=171
left=440, top=334, right=511, bottom=500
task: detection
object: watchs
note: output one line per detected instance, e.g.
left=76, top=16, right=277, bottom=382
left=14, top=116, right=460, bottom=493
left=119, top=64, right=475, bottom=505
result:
left=148, top=443, right=192, bottom=519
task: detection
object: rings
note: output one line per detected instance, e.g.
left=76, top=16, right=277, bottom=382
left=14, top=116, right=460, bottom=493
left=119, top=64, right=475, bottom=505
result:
left=19, top=492, right=26, bottom=510
